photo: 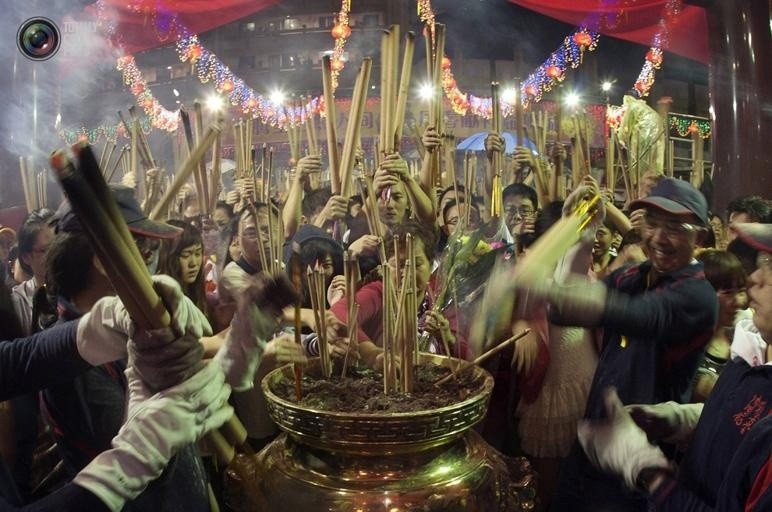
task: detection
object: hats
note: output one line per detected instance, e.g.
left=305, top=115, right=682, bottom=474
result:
left=729, top=221, right=772, bottom=252
left=54, top=182, right=184, bottom=240
left=627, top=177, right=708, bottom=228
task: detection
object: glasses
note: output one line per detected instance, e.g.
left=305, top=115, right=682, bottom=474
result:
left=504, top=205, right=535, bottom=215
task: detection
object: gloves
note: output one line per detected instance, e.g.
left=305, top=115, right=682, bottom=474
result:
left=624, top=400, right=705, bottom=441
left=576, top=384, right=674, bottom=497
left=71, top=274, right=237, bottom=512
left=205, top=272, right=285, bottom=393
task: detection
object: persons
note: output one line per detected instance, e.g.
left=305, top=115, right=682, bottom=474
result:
left=1, top=123, right=771, bottom=512
